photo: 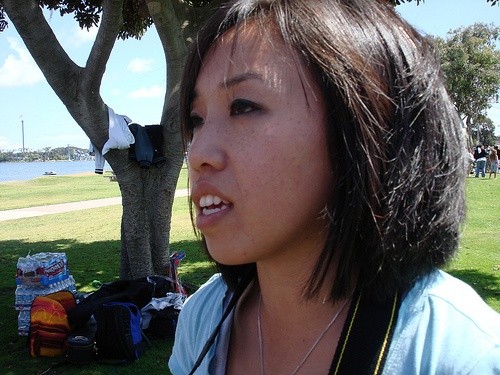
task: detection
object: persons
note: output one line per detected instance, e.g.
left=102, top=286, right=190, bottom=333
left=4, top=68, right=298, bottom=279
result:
left=468, top=142, right=500, bottom=179
left=168, top=0, right=500, bottom=375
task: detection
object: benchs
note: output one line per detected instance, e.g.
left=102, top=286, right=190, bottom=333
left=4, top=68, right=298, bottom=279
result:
left=104, top=175, right=117, bottom=182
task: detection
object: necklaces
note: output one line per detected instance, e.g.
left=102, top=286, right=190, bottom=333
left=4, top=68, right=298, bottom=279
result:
left=256, top=290, right=351, bottom=375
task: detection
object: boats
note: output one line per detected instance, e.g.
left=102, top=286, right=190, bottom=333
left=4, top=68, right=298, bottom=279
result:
left=42, top=171, right=56, bottom=175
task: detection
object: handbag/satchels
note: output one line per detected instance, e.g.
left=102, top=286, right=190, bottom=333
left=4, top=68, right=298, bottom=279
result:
left=29, top=290, right=77, bottom=369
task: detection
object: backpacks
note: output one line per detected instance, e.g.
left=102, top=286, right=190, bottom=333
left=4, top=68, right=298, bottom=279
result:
left=94, top=302, right=144, bottom=362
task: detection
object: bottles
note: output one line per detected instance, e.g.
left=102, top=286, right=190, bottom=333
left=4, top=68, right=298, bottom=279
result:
left=14, top=252, right=77, bottom=337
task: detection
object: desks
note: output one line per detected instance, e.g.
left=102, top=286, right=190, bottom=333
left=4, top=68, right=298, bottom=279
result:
left=106, top=171, right=115, bottom=174
